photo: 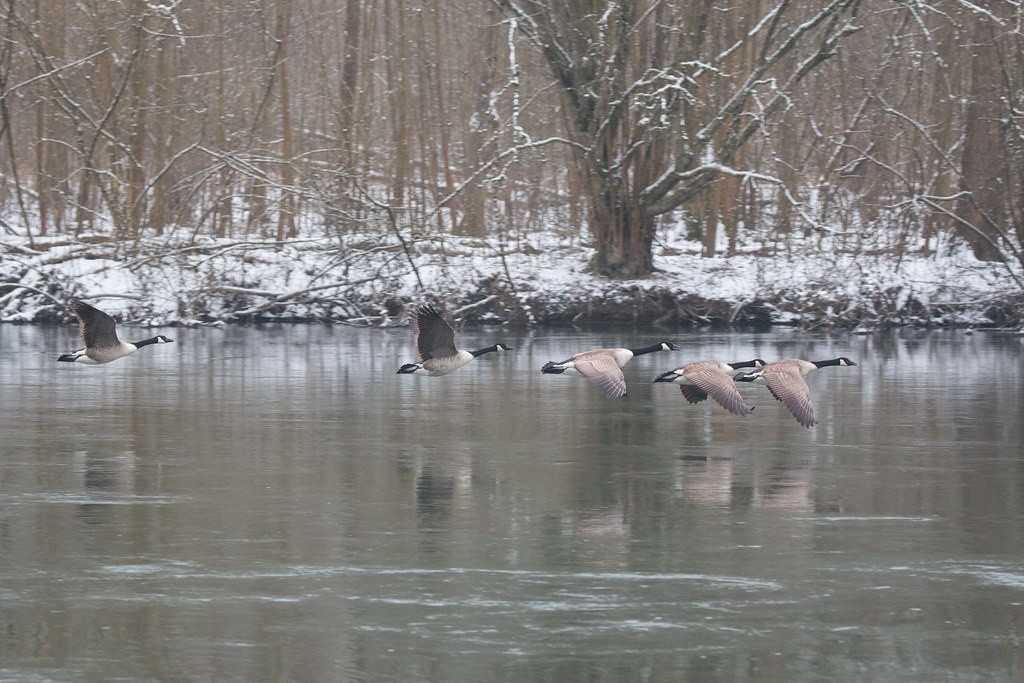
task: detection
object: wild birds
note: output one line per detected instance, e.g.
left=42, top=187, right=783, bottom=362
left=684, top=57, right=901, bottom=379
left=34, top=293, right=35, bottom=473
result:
left=56, top=298, right=174, bottom=365
left=652, top=358, right=768, bottom=417
left=396, top=303, right=514, bottom=377
left=541, top=341, right=683, bottom=400
left=733, top=356, right=857, bottom=429
